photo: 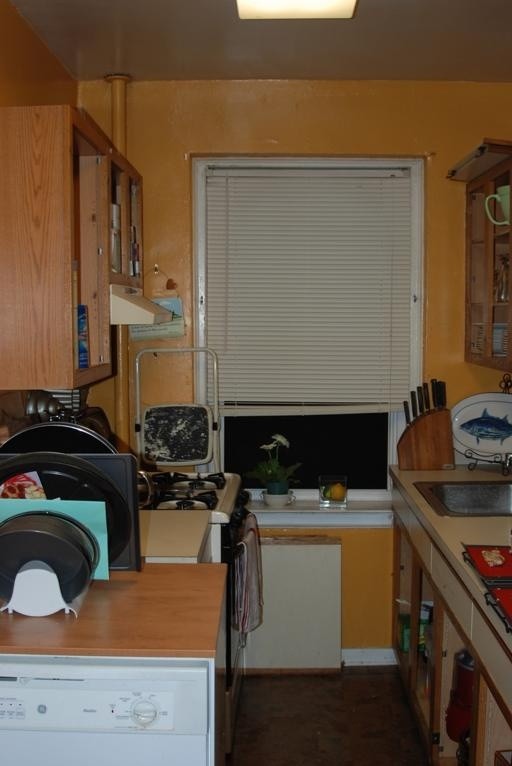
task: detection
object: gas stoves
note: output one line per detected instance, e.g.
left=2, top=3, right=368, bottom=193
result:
left=152, top=471, right=226, bottom=510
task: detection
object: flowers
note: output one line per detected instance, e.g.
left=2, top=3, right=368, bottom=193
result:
left=243, top=433, right=303, bottom=486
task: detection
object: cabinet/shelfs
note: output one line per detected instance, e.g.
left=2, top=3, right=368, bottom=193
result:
left=464, top=154, right=512, bottom=372
left=110, top=147, right=143, bottom=290
left=1, top=105, right=113, bottom=392
left=391, top=483, right=511, bottom=766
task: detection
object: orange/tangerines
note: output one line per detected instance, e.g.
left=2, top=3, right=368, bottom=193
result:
left=324, top=488, right=330, bottom=498
left=332, top=483, right=347, bottom=500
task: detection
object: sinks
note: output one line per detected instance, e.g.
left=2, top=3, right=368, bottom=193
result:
left=413, top=481, right=512, bottom=517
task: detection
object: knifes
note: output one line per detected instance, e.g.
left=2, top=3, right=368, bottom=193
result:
left=403, top=378, right=447, bottom=425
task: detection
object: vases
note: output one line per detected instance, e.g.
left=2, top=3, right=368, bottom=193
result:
left=259, top=482, right=296, bottom=507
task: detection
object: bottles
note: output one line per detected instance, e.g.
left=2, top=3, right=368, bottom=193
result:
left=494, top=254, right=511, bottom=304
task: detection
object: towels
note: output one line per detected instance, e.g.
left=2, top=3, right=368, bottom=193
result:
left=233, top=513, right=264, bottom=634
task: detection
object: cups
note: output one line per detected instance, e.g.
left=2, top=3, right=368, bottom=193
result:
left=318, top=474, right=348, bottom=509
